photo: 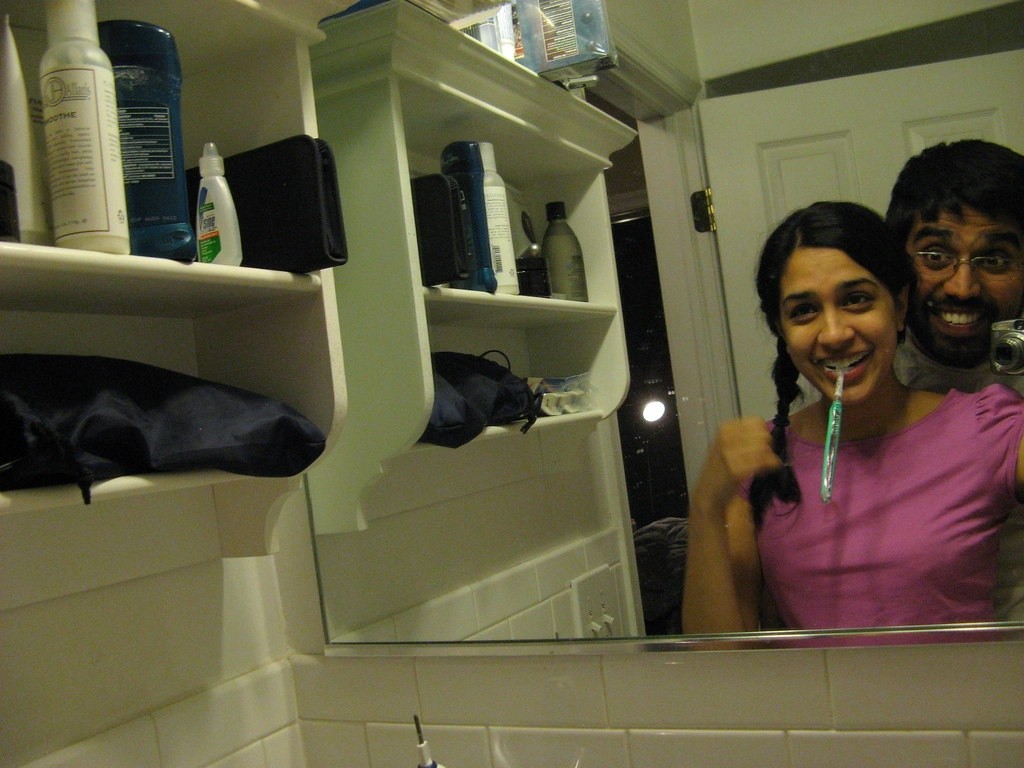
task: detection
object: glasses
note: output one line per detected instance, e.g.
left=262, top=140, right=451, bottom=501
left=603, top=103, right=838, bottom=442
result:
left=906, top=250, right=1024, bottom=283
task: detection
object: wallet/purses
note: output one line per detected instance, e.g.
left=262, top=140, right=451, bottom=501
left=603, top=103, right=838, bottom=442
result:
left=411, top=174, right=472, bottom=287
left=185, top=134, right=348, bottom=275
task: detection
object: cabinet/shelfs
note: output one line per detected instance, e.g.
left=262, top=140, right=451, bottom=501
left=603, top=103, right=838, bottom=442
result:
left=310, top=0, right=640, bottom=531
left=0, top=0, right=348, bottom=516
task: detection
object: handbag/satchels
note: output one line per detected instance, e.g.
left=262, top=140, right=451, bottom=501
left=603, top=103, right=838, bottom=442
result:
left=422, top=348, right=538, bottom=448
left=0, top=353, right=326, bottom=505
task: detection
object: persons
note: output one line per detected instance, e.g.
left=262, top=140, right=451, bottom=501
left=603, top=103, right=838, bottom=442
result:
left=682, top=135, right=1024, bottom=636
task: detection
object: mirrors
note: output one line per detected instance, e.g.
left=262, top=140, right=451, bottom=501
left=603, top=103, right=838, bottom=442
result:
left=305, top=0, right=1024, bottom=657
